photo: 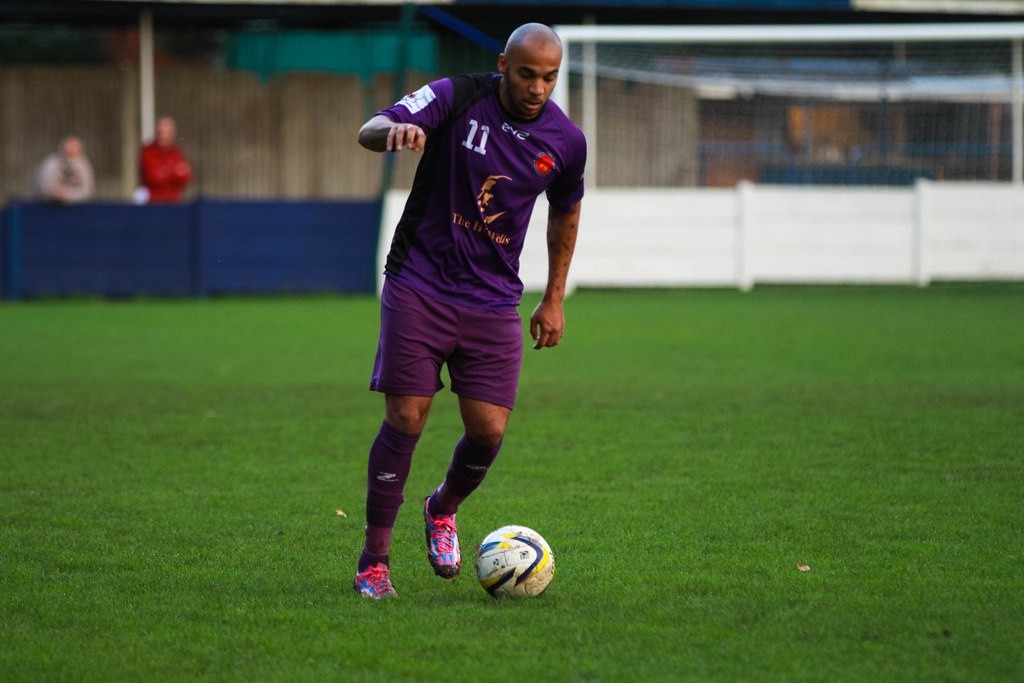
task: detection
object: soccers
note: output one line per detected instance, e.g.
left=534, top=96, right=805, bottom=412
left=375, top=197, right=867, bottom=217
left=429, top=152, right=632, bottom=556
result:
left=476, top=523, right=555, bottom=599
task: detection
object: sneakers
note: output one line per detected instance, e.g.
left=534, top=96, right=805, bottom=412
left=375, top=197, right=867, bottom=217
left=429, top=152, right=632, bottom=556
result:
left=351, top=563, right=398, bottom=601
left=422, top=495, right=461, bottom=579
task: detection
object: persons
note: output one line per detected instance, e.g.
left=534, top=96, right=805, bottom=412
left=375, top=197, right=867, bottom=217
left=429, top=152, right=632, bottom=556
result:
left=351, top=22, right=588, bottom=600
left=22, top=136, right=94, bottom=205
left=139, top=116, right=192, bottom=204
